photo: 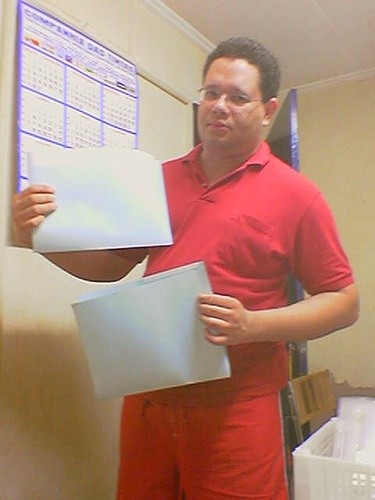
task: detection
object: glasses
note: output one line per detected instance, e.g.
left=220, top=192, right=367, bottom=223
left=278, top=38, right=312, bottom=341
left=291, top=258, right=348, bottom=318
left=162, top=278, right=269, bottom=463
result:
left=198, top=88, right=268, bottom=106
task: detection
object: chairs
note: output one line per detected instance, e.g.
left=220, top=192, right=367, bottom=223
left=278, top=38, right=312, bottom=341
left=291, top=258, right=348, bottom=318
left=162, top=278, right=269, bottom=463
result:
left=289, top=370, right=337, bottom=444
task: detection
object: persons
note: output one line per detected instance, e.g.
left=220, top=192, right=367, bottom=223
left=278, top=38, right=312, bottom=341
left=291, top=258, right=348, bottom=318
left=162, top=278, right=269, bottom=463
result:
left=10, top=37, right=362, bottom=500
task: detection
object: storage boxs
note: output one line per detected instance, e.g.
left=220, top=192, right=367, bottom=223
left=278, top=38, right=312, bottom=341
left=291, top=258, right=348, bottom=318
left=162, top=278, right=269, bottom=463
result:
left=292, top=417, right=375, bottom=499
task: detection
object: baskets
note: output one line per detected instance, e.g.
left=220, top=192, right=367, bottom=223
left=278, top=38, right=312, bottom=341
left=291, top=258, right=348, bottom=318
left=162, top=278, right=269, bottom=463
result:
left=292, top=416, right=375, bottom=500
